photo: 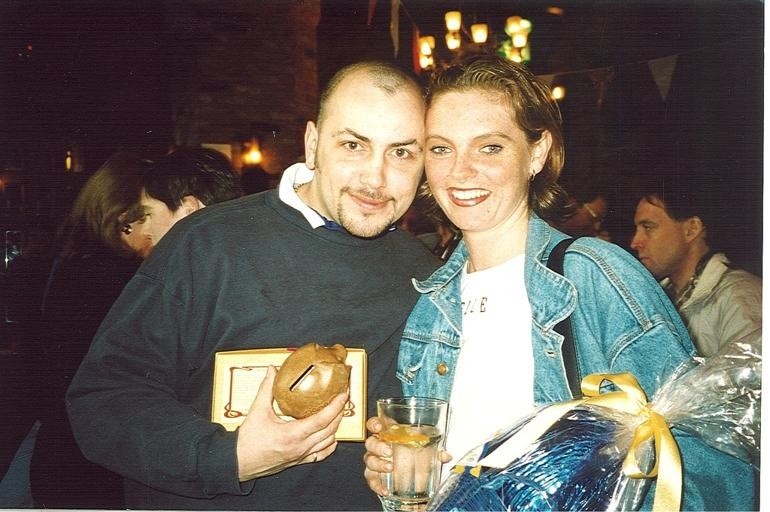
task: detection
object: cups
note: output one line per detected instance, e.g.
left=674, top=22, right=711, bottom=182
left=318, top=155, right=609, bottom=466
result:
left=375, top=396, right=449, bottom=512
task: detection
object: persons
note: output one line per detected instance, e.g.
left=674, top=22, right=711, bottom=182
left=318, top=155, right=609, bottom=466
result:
left=361, top=53, right=758, bottom=512
left=538, top=163, right=615, bottom=240
left=30, top=144, right=247, bottom=510
left=630, top=168, right=762, bottom=375
left=64, top=59, right=447, bottom=512
